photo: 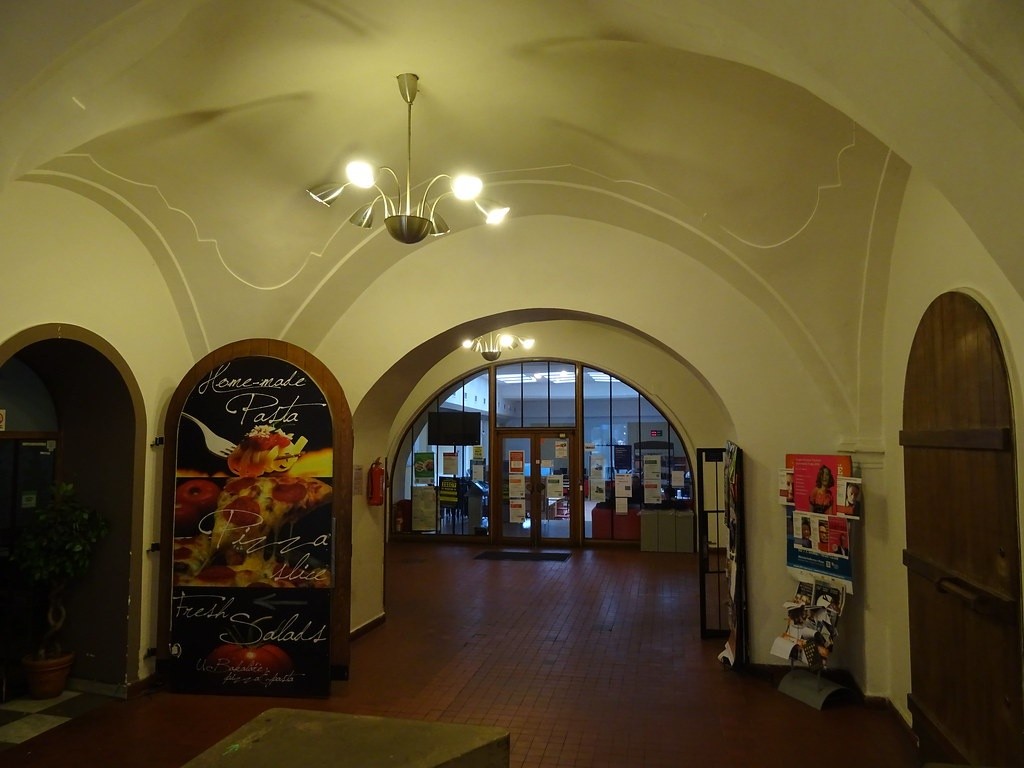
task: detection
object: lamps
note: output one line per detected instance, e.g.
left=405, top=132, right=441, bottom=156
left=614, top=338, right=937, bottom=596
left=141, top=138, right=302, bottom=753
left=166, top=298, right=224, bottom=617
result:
left=306, top=73, right=511, bottom=244
left=463, top=331, right=535, bottom=362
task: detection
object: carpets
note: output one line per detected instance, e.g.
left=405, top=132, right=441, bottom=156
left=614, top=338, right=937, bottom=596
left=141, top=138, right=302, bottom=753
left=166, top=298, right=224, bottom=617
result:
left=473, top=550, right=572, bottom=562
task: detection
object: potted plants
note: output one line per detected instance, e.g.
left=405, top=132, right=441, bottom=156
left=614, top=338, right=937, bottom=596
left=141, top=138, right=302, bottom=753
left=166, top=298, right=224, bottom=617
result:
left=7, top=479, right=111, bottom=698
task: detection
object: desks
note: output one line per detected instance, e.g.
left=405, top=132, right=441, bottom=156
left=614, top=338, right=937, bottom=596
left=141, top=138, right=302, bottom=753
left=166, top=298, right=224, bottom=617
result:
left=180, top=708, right=511, bottom=768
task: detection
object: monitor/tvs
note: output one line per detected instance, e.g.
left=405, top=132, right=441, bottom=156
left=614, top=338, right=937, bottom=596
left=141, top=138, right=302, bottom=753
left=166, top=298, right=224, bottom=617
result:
left=428, top=412, right=481, bottom=446
left=475, top=482, right=486, bottom=490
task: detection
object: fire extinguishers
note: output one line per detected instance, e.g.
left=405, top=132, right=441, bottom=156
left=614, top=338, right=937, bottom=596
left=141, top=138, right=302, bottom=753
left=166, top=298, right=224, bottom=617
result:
left=367, top=456, right=385, bottom=506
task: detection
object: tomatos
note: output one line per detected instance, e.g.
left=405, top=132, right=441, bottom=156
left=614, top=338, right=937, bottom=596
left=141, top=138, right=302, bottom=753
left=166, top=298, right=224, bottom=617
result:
left=175, top=479, right=220, bottom=525
left=208, top=644, right=290, bottom=680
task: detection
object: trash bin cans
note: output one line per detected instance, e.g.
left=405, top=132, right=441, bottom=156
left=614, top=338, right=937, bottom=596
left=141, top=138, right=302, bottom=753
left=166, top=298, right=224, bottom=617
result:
left=675, top=510, right=694, bottom=553
left=640, top=510, right=658, bottom=552
left=658, top=509, right=676, bottom=552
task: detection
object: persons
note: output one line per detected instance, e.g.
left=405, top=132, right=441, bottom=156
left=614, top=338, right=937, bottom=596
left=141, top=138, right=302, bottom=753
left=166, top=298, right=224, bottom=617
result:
left=802, top=522, right=812, bottom=548
left=836, top=534, right=848, bottom=556
left=809, top=465, right=834, bottom=514
left=845, top=483, right=861, bottom=507
left=819, top=524, right=826, bottom=542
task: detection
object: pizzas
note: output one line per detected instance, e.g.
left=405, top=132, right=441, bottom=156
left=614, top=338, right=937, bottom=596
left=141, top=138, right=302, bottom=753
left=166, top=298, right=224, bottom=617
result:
left=185, top=553, right=330, bottom=588
left=174, top=533, right=212, bottom=588
left=210, top=476, right=333, bottom=555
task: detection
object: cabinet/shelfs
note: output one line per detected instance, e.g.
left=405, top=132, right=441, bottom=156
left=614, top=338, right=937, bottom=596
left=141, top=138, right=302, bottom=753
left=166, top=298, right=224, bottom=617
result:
left=641, top=510, right=694, bottom=553
